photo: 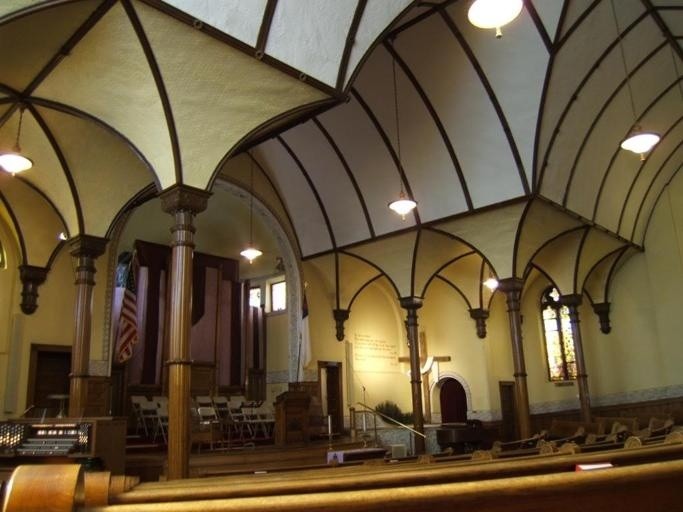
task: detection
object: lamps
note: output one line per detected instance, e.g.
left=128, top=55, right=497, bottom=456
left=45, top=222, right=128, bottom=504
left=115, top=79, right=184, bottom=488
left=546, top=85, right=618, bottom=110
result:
left=0, top=103, right=33, bottom=177
left=610, top=1, right=661, bottom=161
left=239, top=146, right=263, bottom=265
left=387, top=40, right=417, bottom=223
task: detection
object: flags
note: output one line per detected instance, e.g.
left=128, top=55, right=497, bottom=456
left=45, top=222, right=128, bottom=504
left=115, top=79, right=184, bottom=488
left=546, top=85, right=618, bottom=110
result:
left=301, top=294, right=313, bottom=371
left=117, top=263, right=140, bottom=364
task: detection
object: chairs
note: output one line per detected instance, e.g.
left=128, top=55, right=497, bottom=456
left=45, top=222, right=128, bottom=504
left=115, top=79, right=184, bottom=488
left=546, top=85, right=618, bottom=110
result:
left=130, top=394, right=274, bottom=452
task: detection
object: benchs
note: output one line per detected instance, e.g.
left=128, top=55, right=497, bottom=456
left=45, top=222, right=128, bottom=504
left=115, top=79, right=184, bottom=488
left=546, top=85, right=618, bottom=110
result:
left=0, top=416, right=681, bottom=510
